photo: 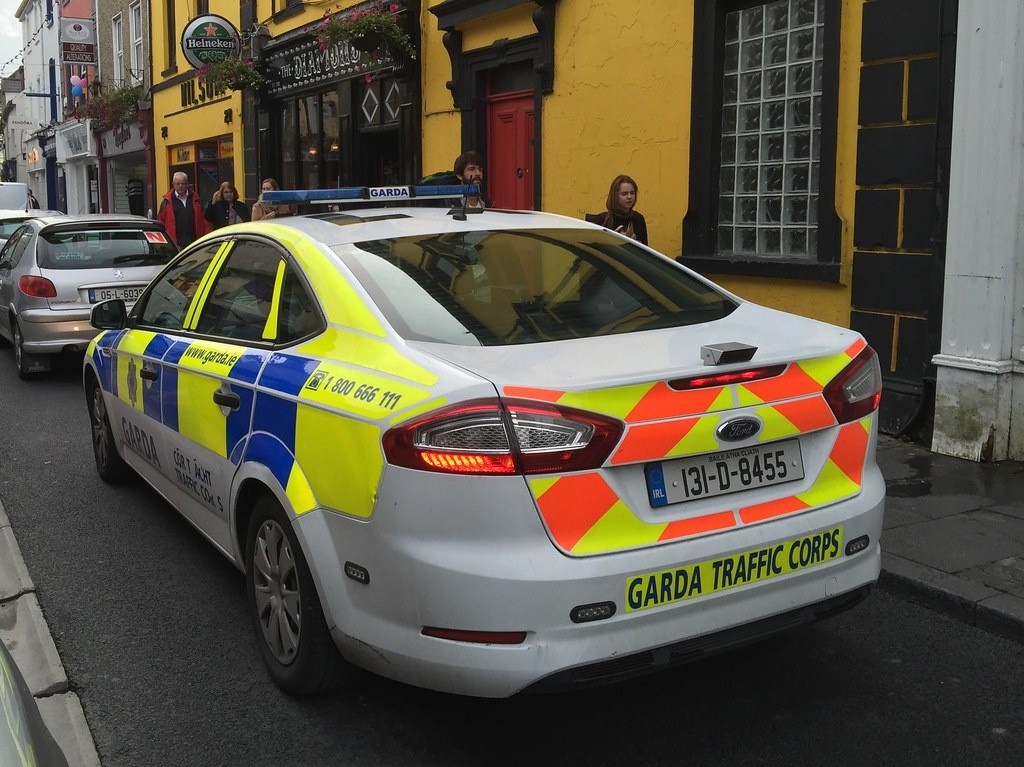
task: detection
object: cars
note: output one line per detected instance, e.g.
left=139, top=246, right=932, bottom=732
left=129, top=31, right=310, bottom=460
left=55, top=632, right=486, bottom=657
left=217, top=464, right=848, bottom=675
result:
left=0, top=208, right=66, bottom=254
left=0, top=214, right=183, bottom=381
left=83, top=183, right=891, bottom=699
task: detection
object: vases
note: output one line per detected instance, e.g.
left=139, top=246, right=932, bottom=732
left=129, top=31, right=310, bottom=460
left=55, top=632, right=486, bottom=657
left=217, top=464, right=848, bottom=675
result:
left=226, top=82, right=249, bottom=90
left=349, top=36, right=382, bottom=52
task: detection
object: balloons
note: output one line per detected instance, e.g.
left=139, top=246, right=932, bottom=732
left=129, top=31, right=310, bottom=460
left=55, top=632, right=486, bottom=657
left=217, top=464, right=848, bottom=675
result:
left=70, top=75, right=87, bottom=96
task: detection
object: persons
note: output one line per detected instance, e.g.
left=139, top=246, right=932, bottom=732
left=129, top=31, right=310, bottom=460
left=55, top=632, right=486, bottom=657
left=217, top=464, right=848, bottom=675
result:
left=156, top=171, right=213, bottom=252
left=415, top=151, right=494, bottom=208
left=204, top=181, right=251, bottom=231
left=252, top=178, right=293, bottom=222
left=593, top=174, right=648, bottom=246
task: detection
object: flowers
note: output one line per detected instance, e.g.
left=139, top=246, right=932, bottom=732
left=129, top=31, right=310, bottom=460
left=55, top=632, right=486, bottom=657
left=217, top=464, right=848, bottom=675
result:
left=303, top=1, right=418, bottom=84
left=194, top=53, right=266, bottom=97
